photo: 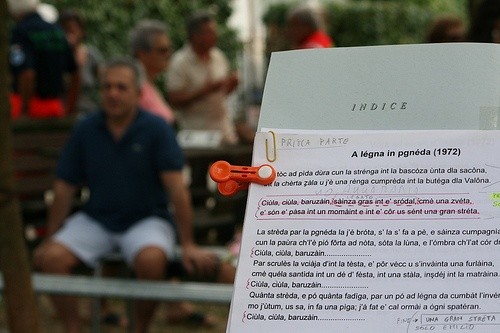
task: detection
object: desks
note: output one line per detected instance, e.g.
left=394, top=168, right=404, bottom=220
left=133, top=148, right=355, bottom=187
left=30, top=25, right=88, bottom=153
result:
left=11, top=133, right=252, bottom=247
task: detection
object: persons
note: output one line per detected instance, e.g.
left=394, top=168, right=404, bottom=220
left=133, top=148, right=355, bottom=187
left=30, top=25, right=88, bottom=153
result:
left=262, top=4, right=295, bottom=55
left=426, top=16, right=463, bottom=42
left=5, top=0, right=103, bottom=244
left=125, top=7, right=240, bottom=247
left=289, top=6, right=335, bottom=50
left=31, top=61, right=215, bottom=333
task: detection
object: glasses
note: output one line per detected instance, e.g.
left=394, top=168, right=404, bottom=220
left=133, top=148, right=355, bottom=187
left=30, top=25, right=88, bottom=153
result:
left=103, top=80, right=136, bottom=92
left=152, top=45, right=170, bottom=54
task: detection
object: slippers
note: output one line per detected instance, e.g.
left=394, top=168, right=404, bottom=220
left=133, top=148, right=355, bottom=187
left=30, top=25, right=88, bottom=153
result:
left=166, top=309, right=218, bottom=327
